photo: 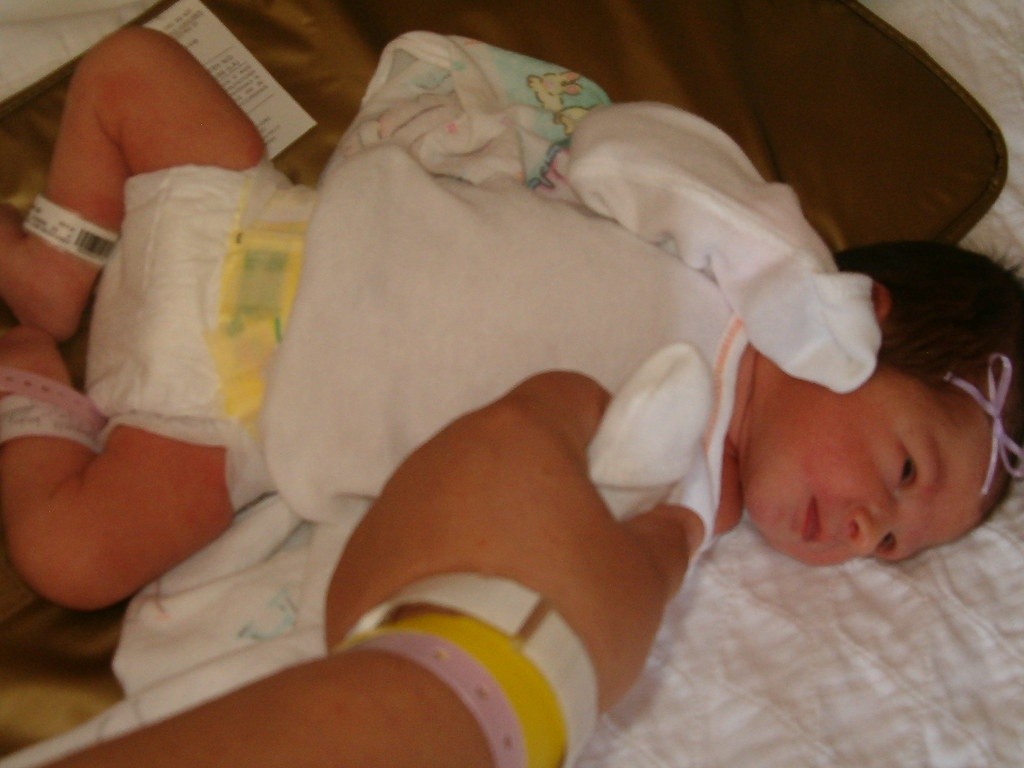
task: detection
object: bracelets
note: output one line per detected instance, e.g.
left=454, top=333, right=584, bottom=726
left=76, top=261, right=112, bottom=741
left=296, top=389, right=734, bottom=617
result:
left=337, top=575, right=607, bottom=766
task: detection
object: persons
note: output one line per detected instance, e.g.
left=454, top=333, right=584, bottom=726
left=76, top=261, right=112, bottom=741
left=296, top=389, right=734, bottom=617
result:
left=28, top=365, right=715, bottom=766
left=2, top=28, right=1021, bottom=613
